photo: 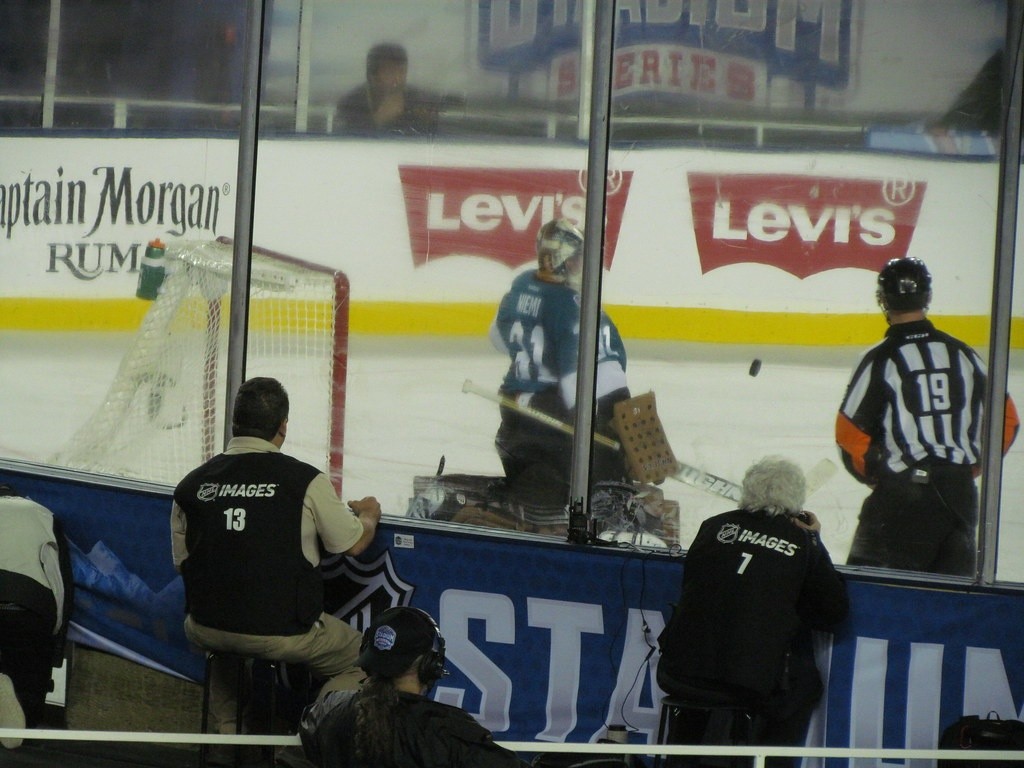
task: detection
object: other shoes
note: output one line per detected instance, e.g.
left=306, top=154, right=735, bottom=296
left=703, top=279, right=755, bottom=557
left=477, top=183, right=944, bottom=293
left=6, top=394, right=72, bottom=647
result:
left=0, top=672, right=25, bottom=750
left=275, top=744, right=318, bottom=768
left=214, top=745, right=264, bottom=766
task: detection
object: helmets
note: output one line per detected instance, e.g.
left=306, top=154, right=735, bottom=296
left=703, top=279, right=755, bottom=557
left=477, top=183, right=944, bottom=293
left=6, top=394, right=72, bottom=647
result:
left=535, top=219, right=584, bottom=283
left=877, top=257, right=932, bottom=311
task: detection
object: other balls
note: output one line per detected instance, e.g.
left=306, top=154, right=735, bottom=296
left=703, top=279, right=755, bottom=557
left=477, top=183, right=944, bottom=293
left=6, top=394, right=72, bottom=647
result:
left=748, top=357, right=762, bottom=376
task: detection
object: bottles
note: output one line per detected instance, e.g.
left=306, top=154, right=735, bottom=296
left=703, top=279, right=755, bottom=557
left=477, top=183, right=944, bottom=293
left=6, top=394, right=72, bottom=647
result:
left=136, top=238, right=165, bottom=302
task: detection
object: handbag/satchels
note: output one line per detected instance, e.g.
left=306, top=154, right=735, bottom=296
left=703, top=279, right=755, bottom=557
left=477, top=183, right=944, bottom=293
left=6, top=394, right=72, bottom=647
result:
left=936, top=711, right=1024, bottom=768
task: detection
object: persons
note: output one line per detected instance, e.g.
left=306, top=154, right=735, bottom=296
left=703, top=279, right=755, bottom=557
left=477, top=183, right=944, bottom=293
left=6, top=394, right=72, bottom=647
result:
left=339, top=41, right=438, bottom=133
left=0, top=485, right=71, bottom=750
left=836, top=257, right=1021, bottom=577
left=655, top=455, right=847, bottom=767
left=172, top=376, right=384, bottom=734
left=311, top=606, right=519, bottom=768
left=410, top=216, right=667, bottom=547
left=934, top=47, right=1004, bottom=133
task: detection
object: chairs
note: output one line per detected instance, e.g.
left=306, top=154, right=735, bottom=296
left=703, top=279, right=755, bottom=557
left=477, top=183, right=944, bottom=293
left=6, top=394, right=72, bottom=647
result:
left=0, top=482, right=75, bottom=728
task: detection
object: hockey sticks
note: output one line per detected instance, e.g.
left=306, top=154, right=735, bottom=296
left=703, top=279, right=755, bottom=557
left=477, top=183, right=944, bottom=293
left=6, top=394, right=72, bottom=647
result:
left=460, top=377, right=839, bottom=504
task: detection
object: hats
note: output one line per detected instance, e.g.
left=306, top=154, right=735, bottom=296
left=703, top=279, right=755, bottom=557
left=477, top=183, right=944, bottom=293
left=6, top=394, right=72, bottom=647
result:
left=355, top=608, right=436, bottom=678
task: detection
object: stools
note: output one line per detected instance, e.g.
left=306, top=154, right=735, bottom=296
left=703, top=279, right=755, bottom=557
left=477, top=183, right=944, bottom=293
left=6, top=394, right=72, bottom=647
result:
left=198, top=651, right=310, bottom=768
left=651, top=695, right=752, bottom=768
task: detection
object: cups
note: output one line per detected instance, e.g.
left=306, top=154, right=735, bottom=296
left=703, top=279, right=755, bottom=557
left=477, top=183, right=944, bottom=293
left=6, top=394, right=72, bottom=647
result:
left=607, top=724, right=627, bottom=744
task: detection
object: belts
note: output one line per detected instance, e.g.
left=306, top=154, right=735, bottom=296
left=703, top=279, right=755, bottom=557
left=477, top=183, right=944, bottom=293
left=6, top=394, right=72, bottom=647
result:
left=0, top=600, right=27, bottom=611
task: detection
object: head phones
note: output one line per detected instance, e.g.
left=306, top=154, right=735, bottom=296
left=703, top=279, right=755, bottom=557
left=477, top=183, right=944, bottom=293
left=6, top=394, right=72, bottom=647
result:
left=358, top=606, right=445, bottom=688
